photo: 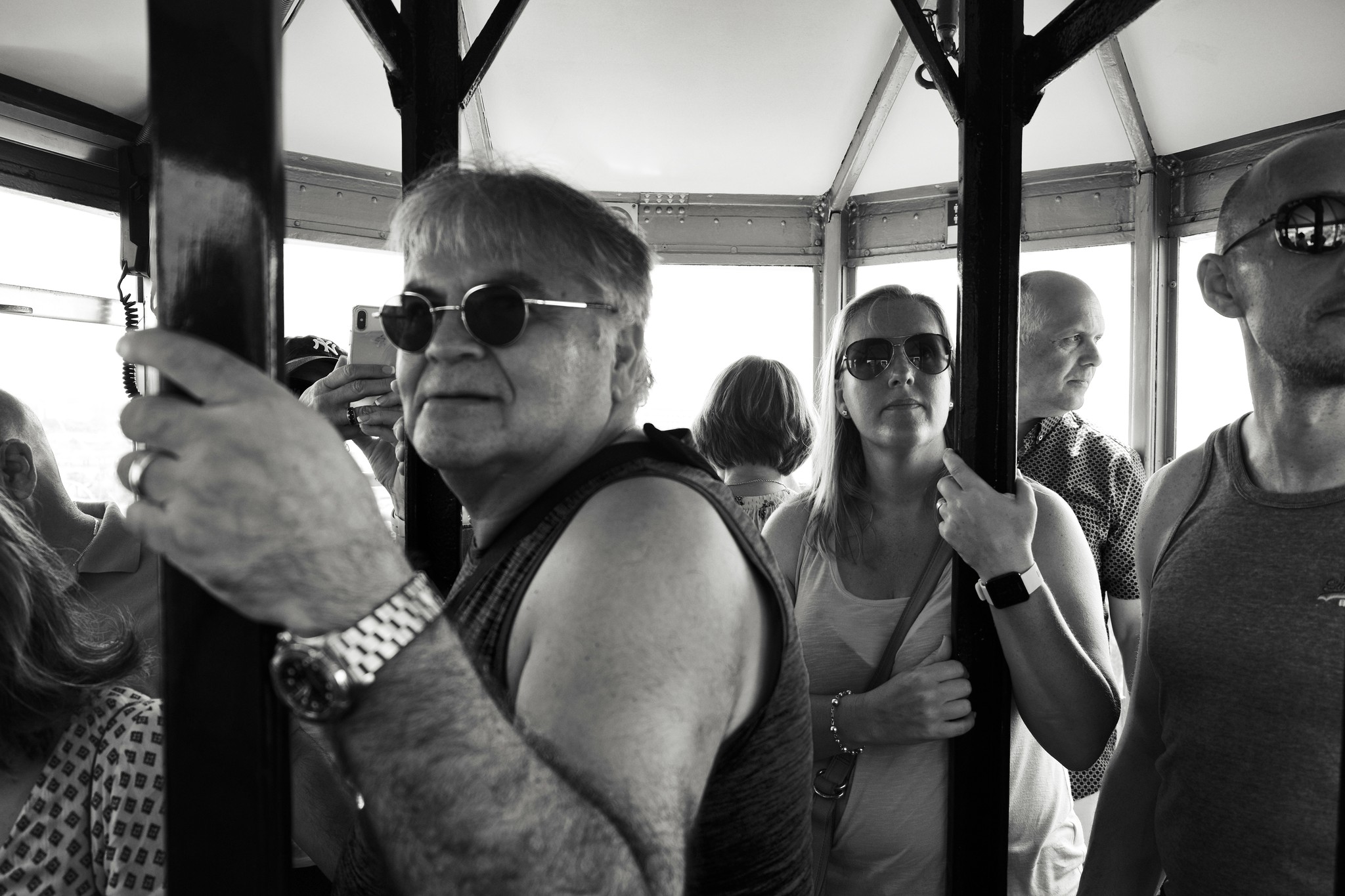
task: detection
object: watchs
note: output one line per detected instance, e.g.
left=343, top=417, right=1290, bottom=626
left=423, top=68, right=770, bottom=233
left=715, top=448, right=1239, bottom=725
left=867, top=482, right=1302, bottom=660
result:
left=268, top=565, right=447, bottom=725
left=975, top=564, right=1043, bottom=610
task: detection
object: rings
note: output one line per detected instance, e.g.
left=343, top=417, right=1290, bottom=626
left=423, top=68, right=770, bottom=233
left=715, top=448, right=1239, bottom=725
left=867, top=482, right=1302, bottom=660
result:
left=128, top=446, right=177, bottom=495
left=346, top=405, right=359, bottom=427
left=936, top=499, right=947, bottom=513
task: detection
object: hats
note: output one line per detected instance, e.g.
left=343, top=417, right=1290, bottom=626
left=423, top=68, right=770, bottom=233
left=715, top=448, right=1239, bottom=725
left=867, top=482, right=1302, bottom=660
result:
left=284, top=333, right=346, bottom=374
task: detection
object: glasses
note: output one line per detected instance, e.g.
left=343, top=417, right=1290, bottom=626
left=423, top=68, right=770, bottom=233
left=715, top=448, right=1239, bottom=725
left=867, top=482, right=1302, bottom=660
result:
left=835, top=334, right=955, bottom=381
left=373, top=283, right=618, bottom=354
left=1223, top=190, right=1345, bottom=256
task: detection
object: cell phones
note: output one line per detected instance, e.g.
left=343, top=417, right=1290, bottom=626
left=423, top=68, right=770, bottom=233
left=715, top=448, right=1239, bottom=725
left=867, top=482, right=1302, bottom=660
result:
left=348, top=305, right=397, bottom=408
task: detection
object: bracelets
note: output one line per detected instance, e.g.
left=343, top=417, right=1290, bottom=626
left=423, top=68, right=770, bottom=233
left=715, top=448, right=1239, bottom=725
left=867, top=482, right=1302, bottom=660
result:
left=829, top=690, right=865, bottom=755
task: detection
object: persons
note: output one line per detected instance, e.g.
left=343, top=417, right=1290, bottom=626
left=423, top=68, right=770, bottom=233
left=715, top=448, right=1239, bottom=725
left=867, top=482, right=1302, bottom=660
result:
left=4, top=119, right=1157, bottom=896
left=1075, top=129, right=1344, bottom=894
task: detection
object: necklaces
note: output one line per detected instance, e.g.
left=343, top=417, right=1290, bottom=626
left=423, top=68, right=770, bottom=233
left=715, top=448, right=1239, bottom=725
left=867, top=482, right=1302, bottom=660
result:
left=69, top=513, right=99, bottom=569
left=724, top=479, right=787, bottom=490
